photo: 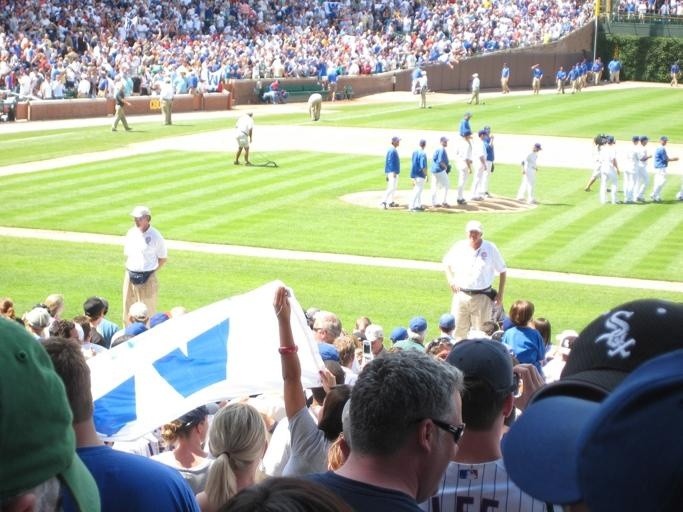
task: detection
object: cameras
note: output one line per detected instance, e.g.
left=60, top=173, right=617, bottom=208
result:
left=512, top=373, right=520, bottom=395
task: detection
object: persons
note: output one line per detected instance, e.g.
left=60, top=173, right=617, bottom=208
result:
left=581, top=130, right=683, bottom=204
left=671, top=61, right=680, bottom=87
left=407, top=138, right=428, bottom=211
left=231, top=113, right=252, bottom=166
left=430, top=137, right=449, bottom=206
left=160, top=79, right=174, bottom=125
left=612, top=1, right=683, bottom=24
left=307, top=92, right=322, bottom=121
left=121, top=206, right=165, bottom=316
left=608, top=56, right=622, bottom=84
left=0, top=1, right=604, bottom=104
left=470, top=131, right=488, bottom=202
left=441, top=221, right=507, bottom=332
left=418, top=70, right=428, bottom=108
left=112, top=81, right=132, bottom=130
left=380, top=137, right=401, bottom=211
left=0, top=287, right=682, bottom=512
left=483, top=127, right=495, bottom=195
left=514, top=143, right=544, bottom=205
left=453, top=130, right=472, bottom=203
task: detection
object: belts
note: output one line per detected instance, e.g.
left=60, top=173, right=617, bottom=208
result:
left=459, top=285, right=492, bottom=295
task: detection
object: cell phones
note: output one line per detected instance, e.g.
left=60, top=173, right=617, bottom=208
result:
left=362, top=340, right=372, bottom=358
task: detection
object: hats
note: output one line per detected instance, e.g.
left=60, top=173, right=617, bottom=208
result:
left=26, top=307, right=50, bottom=328
left=0, top=315, right=102, bottom=512
left=439, top=313, right=455, bottom=329
left=131, top=206, right=151, bottom=217
left=448, top=337, right=514, bottom=387
left=467, top=220, right=482, bottom=233
left=127, top=302, right=166, bottom=335
left=390, top=316, right=427, bottom=341
left=500, top=296, right=683, bottom=507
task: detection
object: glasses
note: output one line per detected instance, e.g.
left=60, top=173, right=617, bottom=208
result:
left=412, top=417, right=466, bottom=443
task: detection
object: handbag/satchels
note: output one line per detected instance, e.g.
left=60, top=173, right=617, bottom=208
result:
left=129, top=271, right=149, bottom=283
